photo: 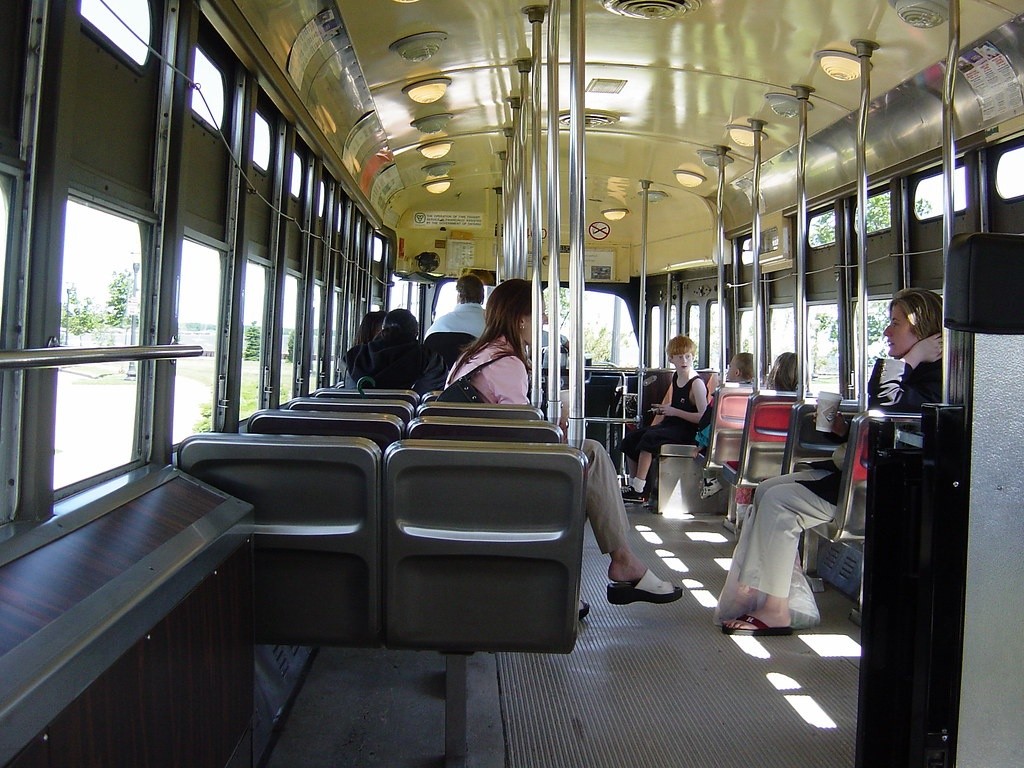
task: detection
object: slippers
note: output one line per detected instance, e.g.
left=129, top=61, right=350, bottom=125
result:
left=722, top=614, right=794, bottom=635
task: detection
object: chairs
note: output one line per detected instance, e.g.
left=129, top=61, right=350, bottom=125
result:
left=639, top=369, right=922, bottom=627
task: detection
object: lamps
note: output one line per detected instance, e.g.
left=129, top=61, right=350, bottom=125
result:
left=416, top=140, right=457, bottom=158
left=766, top=89, right=811, bottom=119
left=410, top=114, right=454, bottom=134
left=399, top=76, right=454, bottom=104
left=816, top=46, right=874, bottom=81
left=671, top=168, right=707, bottom=188
left=421, top=161, right=455, bottom=177
left=727, top=123, right=769, bottom=147
left=423, top=177, right=453, bottom=194
left=390, top=30, right=447, bottom=63
left=697, top=148, right=734, bottom=169
left=601, top=209, right=629, bottom=222
left=638, top=191, right=668, bottom=204
left=888, top=1, right=950, bottom=27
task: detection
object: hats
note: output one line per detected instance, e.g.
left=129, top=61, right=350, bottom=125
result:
left=383, top=309, right=419, bottom=337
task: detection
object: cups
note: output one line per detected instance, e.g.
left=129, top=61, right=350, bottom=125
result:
left=816, top=390, right=842, bottom=433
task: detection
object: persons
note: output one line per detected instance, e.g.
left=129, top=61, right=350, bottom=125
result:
left=445, top=279, right=683, bottom=620
left=693, top=353, right=754, bottom=500
left=423, top=275, right=487, bottom=343
left=619, top=335, right=708, bottom=504
left=337, top=309, right=449, bottom=399
left=722, top=287, right=943, bottom=636
left=736, top=352, right=798, bottom=530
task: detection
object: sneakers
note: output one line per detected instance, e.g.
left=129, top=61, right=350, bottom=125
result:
left=621, top=486, right=644, bottom=501
left=699, top=478, right=723, bottom=498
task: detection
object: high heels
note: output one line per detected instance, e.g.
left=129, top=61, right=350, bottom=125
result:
left=578, top=599, right=591, bottom=620
left=607, top=568, right=683, bottom=605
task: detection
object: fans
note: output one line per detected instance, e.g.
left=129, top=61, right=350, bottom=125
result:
left=414, top=250, right=441, bottom=274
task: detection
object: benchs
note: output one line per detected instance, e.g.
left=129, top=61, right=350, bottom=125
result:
left=177, top=388, right=590, bottom=768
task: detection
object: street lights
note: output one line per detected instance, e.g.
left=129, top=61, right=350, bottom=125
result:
left=64, top=289, right=71, bottom=344
left=125, top=252, right=139, bottom=379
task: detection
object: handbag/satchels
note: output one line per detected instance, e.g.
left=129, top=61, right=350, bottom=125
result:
left=437, top=376, right=483, bottom=402
left=715, top=503, right=821, bottom=628
